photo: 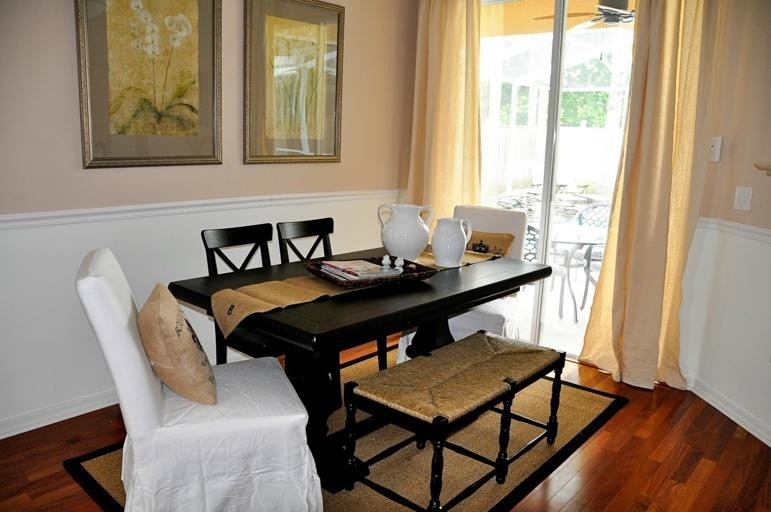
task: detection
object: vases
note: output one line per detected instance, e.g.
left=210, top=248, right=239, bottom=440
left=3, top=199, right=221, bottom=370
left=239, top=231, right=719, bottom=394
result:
left=366, top=204, right=434, bottom=264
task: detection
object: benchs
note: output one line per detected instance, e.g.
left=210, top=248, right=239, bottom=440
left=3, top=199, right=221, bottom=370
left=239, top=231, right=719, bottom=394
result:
left=342, top=325, right=570, bottom=512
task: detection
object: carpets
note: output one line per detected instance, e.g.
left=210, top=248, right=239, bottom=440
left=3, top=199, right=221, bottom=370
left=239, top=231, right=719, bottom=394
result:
left=61, top=323, right=631, bottom=512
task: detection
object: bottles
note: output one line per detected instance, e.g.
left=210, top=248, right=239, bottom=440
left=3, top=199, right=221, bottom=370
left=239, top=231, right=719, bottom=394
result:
left=378, top=204, right=435, bottom=263
left=475, top=239, right=487, bottom=253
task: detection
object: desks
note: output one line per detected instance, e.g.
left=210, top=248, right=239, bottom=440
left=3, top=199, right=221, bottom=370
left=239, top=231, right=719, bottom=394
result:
left=547, top=217, right=611, bottom=324
left=167, top=237, right=555, bottom=489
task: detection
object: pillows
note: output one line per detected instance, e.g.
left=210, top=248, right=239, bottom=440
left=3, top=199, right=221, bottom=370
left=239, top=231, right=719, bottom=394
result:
left=137, top=281, right=220, bottom=407
left=463, top=228, right=515, bottom=259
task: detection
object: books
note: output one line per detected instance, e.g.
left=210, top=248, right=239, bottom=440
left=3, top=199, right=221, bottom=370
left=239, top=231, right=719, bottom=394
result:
left=320, top=260, right=397, bottom=282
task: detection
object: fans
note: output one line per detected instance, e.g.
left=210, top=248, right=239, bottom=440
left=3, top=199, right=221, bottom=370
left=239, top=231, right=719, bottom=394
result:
left=531, top=0, right=641, bottom=36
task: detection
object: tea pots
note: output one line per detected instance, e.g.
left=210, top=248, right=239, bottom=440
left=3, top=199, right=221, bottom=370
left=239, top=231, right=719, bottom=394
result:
left=430, top=215, right=473, bottom=270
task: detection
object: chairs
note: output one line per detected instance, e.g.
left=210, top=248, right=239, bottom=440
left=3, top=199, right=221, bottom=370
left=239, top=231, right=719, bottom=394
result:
left=523, top=224, right=578, bottom=328
left=397, top=202, right=529, bottom=364
left=72, top=245, right=332, bottom=512
left=275, top=215, right=393, bottom=411
left=197, top=222, right=335, bottom=437
left=569, top=202, right=618, bottom=314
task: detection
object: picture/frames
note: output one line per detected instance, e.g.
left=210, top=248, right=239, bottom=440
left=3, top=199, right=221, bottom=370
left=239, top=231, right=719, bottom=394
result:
left=241, top=0, right=348, bottom=168
left=71, top=1, right=227, bottom=170
